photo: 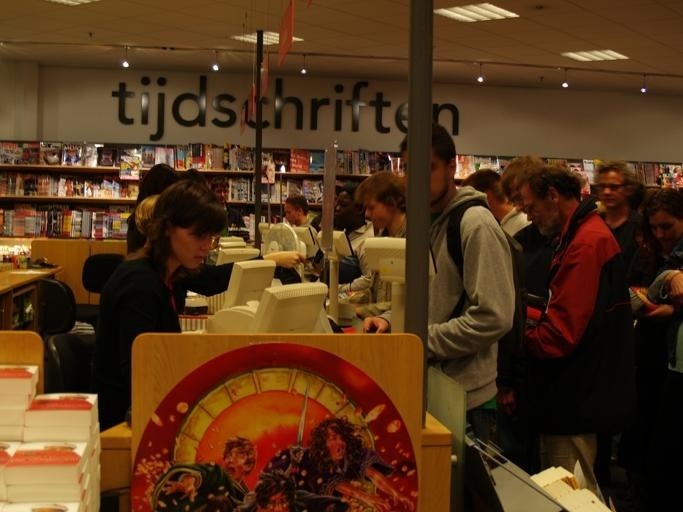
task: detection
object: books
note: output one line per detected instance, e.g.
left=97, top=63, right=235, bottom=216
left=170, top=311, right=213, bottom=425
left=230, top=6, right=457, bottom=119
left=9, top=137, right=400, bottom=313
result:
left=336, top=145, right=406, bottom=175
left=248, top=212, right=282, bottom=242
left=529, top=467, right=610, bottom=512
left=0, top=204, right=135, bottom=240
left=454, top=153, right=681, bottom=188
left=0, top=364, right=102, bottom=512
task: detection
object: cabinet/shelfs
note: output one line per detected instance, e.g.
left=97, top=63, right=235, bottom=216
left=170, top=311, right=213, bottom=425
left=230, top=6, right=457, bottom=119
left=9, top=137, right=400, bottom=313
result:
left=1, top=138, right=522, bottom=243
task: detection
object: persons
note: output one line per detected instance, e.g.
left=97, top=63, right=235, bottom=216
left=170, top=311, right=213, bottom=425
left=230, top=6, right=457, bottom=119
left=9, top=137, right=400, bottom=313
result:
left=514, top=164, right=628, bottom=438
left=97, top=179, right=229, bottom=427
left=632, top=187, right=683, bottom=387
left=459, top=169, right=533, bottom=239
left=594, top=160, right=641, bottom=258
left=500, top=156, right=554, bottom=299
left=308, top=185, right=341, bottom=233
left=362, top=122, right=516, bottom=448
left=126, top=163, right=180, bottom=254
left=284, top=193, right=318, bottom=252
left=129, top=195, right=306, bottom=298
left=331, top=187, right=375, bottom=295
left=352, top=169, right=406, bottom=239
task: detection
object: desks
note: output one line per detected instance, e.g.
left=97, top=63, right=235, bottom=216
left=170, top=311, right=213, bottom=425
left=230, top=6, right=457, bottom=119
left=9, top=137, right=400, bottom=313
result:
left=0, top=261, right=65, bottom=332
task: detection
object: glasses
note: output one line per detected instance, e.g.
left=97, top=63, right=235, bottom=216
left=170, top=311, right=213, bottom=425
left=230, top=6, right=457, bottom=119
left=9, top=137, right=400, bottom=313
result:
left=595, top=182, right=625, bottom=190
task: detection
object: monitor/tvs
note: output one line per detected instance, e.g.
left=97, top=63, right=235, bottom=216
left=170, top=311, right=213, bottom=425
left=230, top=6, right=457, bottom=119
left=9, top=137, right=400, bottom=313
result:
left=216, top=248, right=260, bottom=266
left=224, top=259, right=276, bottom=307
left=251, top=281, right=328, bottom=334
